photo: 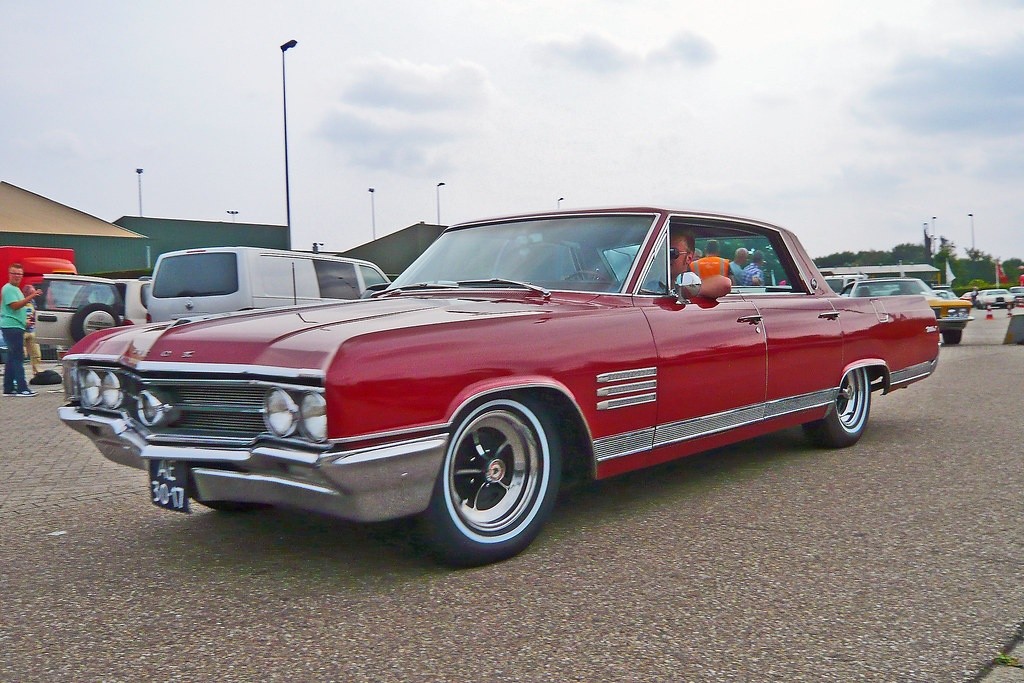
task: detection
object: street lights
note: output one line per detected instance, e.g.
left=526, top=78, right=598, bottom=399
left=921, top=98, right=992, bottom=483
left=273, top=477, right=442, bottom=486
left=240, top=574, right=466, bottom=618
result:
left=279, top=40, right=297, bottom=226
left=932, top=217, right=936, bottom=253
left=368, top=188, right=375, bottom=240
left=136, top=168, right=143, bottom=217
left=437, top=182, right=445, bottom=225
left=968, top=214, right=974, bottom=248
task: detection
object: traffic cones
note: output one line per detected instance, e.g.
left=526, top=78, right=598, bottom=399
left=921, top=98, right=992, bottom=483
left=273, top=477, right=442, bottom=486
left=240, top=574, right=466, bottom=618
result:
left=984, top=302, right=994, bottom=319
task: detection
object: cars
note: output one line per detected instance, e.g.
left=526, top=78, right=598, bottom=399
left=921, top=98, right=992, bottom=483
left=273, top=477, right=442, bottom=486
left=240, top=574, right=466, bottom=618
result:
left=960, top=292, right=979, bottom=301
left=1009, top=286, right=1024, bottom=305
left=975, top=289, right=1015, bottom=310
left=57, top=205, right=941, bottom=563
left=824, top=274, right=868, bottom=296
left=834, top=277, right=975, bottom=344
left=932, top=285, right=958, bottom=299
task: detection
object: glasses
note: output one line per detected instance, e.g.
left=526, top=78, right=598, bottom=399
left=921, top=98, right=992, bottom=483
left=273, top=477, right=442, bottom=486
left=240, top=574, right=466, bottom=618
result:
left=668, top=247, right=689, bottom=259
left=9, top=272, right=24, bottom=276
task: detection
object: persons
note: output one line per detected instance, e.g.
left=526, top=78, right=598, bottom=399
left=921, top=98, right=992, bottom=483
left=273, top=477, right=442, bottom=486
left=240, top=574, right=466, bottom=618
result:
left=0, top=263, right=40, bottom=397
left=642, top=228, right=764, bottom=295
left=1017, top=266, right=1024, bottom=286
left=971, top=287, right=976, bottom=307
left=22, top=285, right=41, bottom=375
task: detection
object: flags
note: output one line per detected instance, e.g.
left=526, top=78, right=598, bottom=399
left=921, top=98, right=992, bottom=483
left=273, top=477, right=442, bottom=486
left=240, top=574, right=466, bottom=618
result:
left=946, top=261, right=956, bottom=285
left=997, top=262, right=1009, bottom=283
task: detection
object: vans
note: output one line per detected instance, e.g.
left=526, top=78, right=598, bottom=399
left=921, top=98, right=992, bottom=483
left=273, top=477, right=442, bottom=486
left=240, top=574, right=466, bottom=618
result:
left=146, top=246, right=392, bottom=324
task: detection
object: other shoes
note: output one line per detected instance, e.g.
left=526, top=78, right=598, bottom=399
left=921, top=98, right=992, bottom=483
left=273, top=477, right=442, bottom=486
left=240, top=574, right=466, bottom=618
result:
left=16, top=388, right=38, bottom=397
left=2, top=389, right=18, bottom=397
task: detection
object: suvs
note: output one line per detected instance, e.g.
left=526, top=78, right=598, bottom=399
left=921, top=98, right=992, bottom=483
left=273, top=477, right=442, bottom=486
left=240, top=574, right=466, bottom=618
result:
left=34, top=274, right=153, bottom=347
